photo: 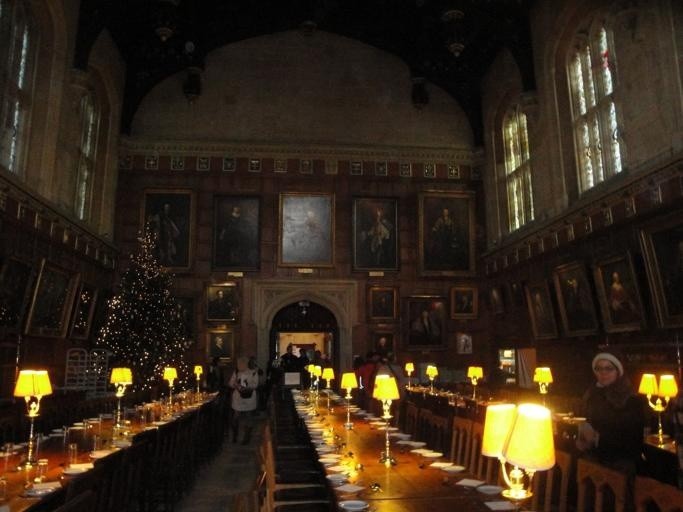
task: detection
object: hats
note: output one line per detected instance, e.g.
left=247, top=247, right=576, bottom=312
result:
left=591, top=346, right=625, bottom=377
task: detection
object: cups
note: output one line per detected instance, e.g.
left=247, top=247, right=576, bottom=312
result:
left=3, top=441, right=13, bottom=457
left=93, top=434, right=102, bottom=450
left=68, top=444, right=77, bottom=466
left=38, top=458, right=48, bottom=482
left=0, top=478, right=8, bottom=501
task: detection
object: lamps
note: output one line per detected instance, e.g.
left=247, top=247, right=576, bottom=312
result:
left=637, top=374, right=678, bottom=448
left=405, top=362, right=414, bottom=390
left=14, top=371, right=53, bottom=468
left=373, top=377, right=401, bottom=466
left=163, top=368, right=177, bottom=405
left=308, top=365, right=357, bottom=429
left=110, top=367, right=133, bottom=428
left=467, top=367, right=483, bottom=401
left=426, top=366, right=438, bottom=393
left=534, top=367, right=553, bottom=405
left=194, top=366, right=203, bottom=404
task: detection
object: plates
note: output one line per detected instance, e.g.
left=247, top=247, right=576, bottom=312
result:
left=293, top=391, right=327, bottom=444
left=400, top=435, right=411, bottom=439
left=378, top=423, right=390, bottom=426
left=325, top=474, right=346, bottom=482
left=443, top=466, right=465, bottom=474
left=422, top=452, right=442, bottom=457
left=325, top=388, right=372, bottom=417
left=318, top=458, right=339, bottom=463
left=88, top=453, right=107, bottom=458
left=339, top=501, right=369, bottom=511
left=26, top=488, right=53, bottom=496
left=155, top=422, right=166, bottom=425
left=478, top=485, right=501, bottom=494
left=145, top=427, right=158, bottom=430
left=64, top=469, right=88, bottom=478
left=412, top=443, right=426, bottom=447
left=390, top=428, right=398, bottom=432
left=315, top=448, right=335, bottom=452
left=371, top=417, right=382, bottom=421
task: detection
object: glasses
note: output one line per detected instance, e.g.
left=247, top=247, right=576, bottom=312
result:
left=594, top=366, right=615, bottom=372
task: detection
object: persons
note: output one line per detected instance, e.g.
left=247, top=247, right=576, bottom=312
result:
left=209, top=289, right=234, bottom=318
left=248, top=355, right=264, bottom=416
left=211, top=356, right=225, bottom=384
left=608, top=271, right=640, bottom=320
left=375, top=337, right=389, bottom=355
left=211, top=336, right=228, bottom=357
left=218, top=203, right=254, bottom=267
left=147, top=200, right=184, bottom=265
left=227, top=355, right=260, bottom=445
left=567, top=278, right=590, bottom=312
left=279, top=345, right=298, bottom=360
left=532, top=292, right=555, bottom=334
left=297, top=349, right=309, bottom=366
left=455, top=294, right=473, bottom=313
left=570, top=343, right=647, bottom=453
left=410, top=302, right=440, bottom=346
left=428, top=207, right=456, bottom=270
left=310, top=350, right=326, bottom=368
left=365, top=207, right=391, bottom=267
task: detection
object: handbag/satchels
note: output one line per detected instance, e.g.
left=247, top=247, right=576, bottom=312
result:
left=239, top=389, right=253, bottom=398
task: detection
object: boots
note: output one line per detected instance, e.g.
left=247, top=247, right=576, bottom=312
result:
left=239, top=427, right=251, bottom=445
left=231, top=426, right=238, bottom=443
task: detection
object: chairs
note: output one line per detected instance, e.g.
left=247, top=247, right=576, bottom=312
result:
left=0, top=387, right=230, bottom=512
